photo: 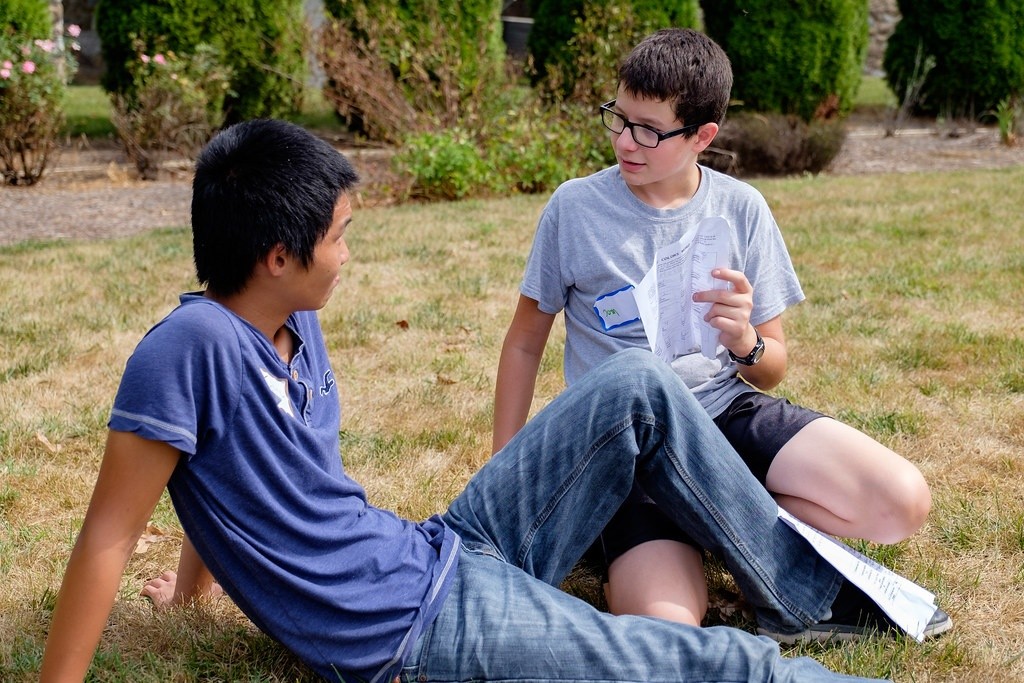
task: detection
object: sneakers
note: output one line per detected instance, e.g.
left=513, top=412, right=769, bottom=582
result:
left=756, top=596, right=953, bottom=646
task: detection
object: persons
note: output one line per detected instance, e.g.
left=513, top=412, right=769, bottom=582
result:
left=492, top=27, right=932, bottom=628
left=37, top=117, right=954, bottom=683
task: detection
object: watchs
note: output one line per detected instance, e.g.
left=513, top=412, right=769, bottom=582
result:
left=727, top=327, right=766, bottom=366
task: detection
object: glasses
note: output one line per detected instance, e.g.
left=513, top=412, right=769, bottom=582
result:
left=598, top=97, right=700, bottom=148
